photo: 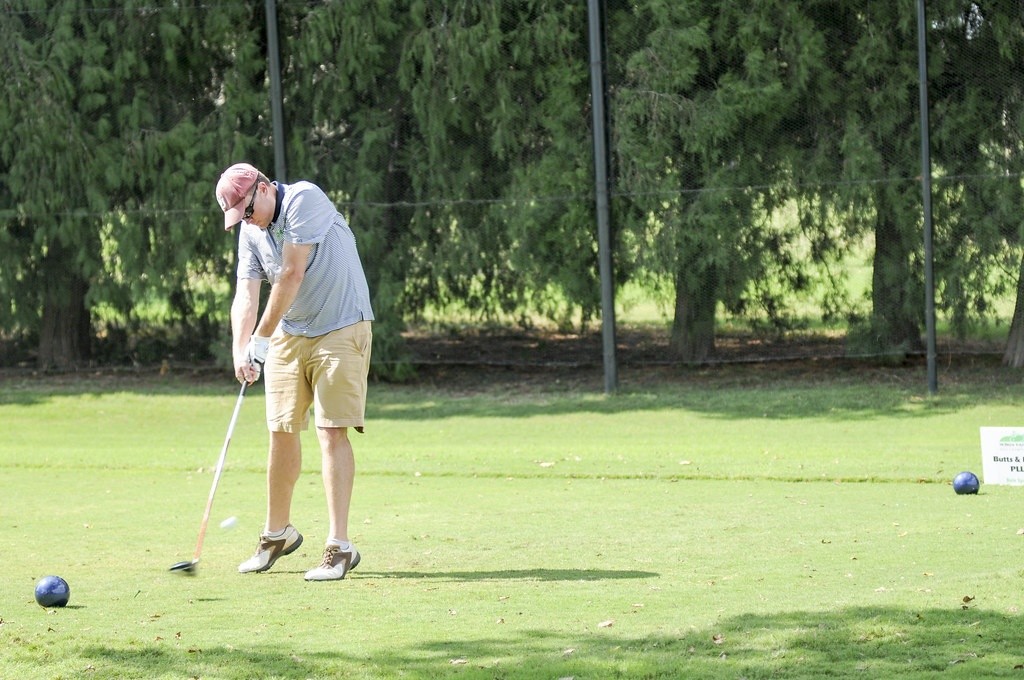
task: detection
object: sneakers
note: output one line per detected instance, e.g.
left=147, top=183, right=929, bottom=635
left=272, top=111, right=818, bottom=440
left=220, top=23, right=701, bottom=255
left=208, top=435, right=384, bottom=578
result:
left=304, top=541, right=361, bottom=581
left=238, top=522, right=303, bottom=575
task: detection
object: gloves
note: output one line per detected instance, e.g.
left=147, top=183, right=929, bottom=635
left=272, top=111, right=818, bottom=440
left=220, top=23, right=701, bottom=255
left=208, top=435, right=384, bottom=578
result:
left=246, top=335, right=270, bottom=387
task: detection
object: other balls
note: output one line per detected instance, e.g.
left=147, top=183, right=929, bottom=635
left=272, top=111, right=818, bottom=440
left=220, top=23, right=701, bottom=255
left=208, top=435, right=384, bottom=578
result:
left=34, top=575, right=71, bottom=608
left=953, top=471, right=979, bottom=495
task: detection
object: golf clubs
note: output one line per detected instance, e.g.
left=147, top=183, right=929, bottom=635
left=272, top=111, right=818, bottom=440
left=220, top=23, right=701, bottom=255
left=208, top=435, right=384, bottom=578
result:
left=164, top=378, right=249, bottom=571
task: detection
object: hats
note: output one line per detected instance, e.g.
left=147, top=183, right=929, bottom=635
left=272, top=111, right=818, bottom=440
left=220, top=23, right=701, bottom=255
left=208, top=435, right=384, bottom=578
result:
left=215, top=162, right=258, bottom=231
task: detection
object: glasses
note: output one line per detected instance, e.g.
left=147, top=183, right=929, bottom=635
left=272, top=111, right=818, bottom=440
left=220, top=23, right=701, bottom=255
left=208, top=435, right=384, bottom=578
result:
left=242, top=180, right=259, bottom=218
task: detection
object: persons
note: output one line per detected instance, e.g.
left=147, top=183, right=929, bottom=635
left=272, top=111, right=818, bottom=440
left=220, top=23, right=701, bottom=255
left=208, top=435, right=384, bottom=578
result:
left=215, top=163, right=375, bottom=580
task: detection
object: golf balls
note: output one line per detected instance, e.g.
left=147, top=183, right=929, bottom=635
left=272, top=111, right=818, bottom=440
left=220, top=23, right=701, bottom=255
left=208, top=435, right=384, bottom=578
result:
left=219, top=516, right=238, bottom=530
